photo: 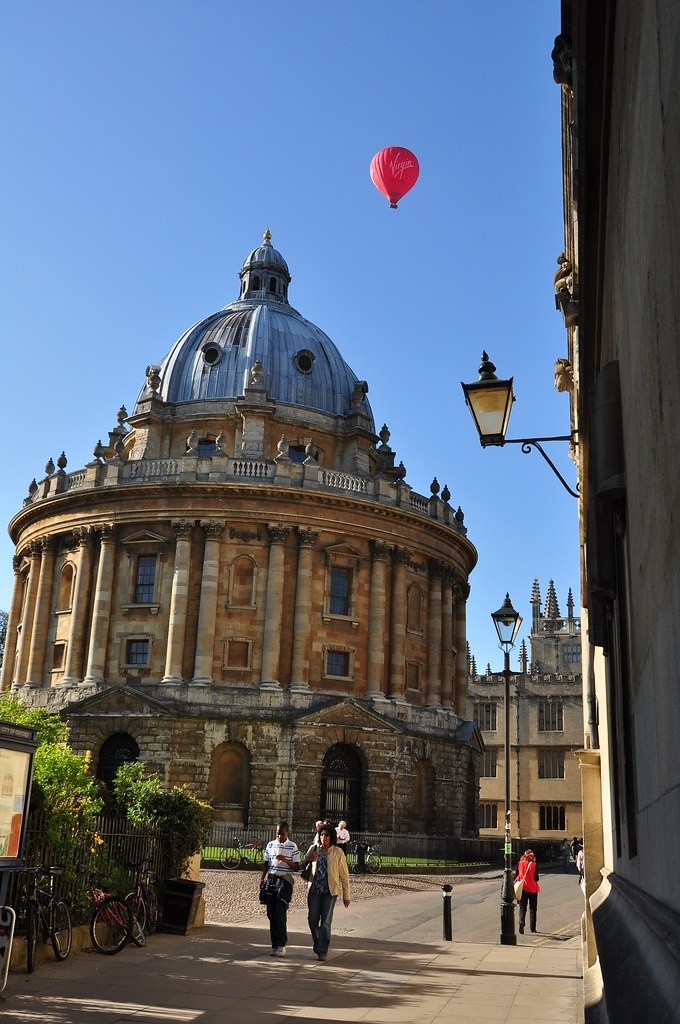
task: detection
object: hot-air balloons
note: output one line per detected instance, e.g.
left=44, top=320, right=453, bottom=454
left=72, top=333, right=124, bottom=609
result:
left=369, top=147, right=419, bottom=209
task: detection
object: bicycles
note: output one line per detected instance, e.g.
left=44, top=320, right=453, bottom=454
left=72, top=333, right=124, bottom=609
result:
left=219, top=835, right=266, bottom=870
left=122, top=858, right=160, bottom=941
left=14, top=865, right=72, bottom=974
left=89, top=869, right=147, bottom=955
left=348, top=840, right=382, bottom=874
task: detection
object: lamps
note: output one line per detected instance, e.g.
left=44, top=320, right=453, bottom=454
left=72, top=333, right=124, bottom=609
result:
left=459, top=351, right=581, bottom=499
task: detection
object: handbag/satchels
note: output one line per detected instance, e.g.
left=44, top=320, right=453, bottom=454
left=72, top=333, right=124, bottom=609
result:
left=259, top=874, right=287, bottom=904
left=513, top=879, right=524, bottom=900
left=301, top=844, right=318, bottom=881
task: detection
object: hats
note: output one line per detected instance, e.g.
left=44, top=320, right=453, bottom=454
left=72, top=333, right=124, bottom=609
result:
left=524, top=849, right=534, bottom=860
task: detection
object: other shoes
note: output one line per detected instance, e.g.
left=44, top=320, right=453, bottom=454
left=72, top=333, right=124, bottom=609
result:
left=277, top=946, right=287, bottom=957
left=530, top=928, right=537, bottom=933
left=519, top=922, right=525, bottom=934
left=318, top=952, right=328, bottom=961
left=270, top=948, right=278, bottom=956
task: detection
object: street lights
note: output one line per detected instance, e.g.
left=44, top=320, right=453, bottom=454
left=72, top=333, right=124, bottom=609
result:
left=490, top=591, right=524, bottom=946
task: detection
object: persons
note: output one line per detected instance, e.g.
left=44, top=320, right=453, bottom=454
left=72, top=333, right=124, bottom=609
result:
left=299, top=824, right=351, bottom=960
left=560, top=836, right=584, bottom=884
left=513, top=849, right=540, bottom=934
left=312, top=818, right=350, bottom=863
left=260, top=822, right=300, bottom=957
